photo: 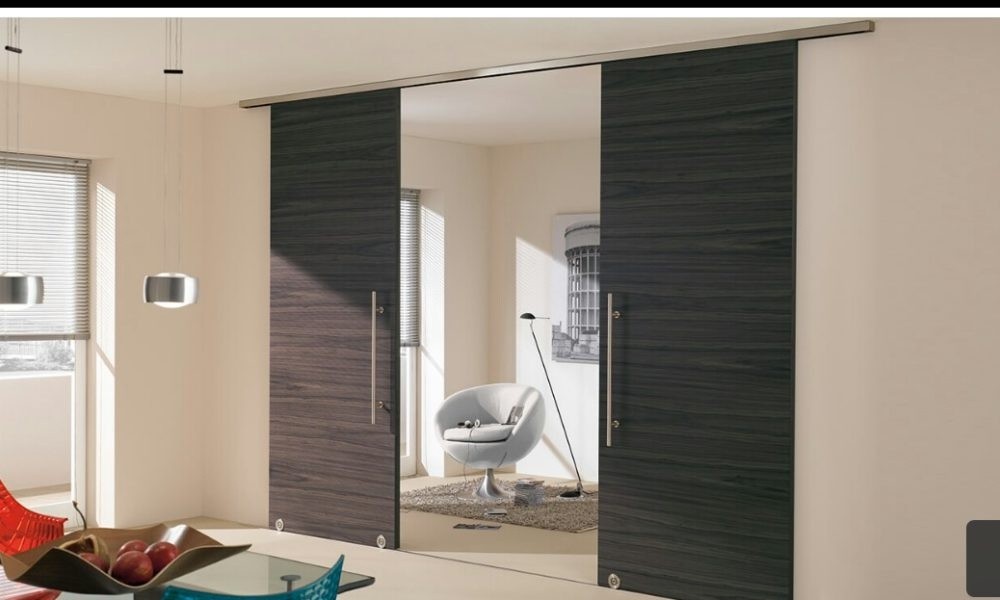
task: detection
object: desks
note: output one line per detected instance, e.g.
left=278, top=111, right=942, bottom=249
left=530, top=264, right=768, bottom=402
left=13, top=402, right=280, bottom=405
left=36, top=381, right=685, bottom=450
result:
left=1, top=550, right=376, bottom=600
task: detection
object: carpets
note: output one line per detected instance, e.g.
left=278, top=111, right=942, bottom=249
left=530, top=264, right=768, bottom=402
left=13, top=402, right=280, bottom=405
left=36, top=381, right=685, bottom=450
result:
left=397, top=477, right=598, bottom=532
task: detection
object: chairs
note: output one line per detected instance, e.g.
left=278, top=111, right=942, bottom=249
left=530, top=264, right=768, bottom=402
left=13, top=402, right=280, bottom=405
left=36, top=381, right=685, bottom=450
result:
left=437, top=383, right=545, bottom=500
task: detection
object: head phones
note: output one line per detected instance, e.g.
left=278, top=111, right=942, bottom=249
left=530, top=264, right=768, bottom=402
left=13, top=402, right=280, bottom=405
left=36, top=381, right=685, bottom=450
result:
left=457, top=419, right=481, bottom=428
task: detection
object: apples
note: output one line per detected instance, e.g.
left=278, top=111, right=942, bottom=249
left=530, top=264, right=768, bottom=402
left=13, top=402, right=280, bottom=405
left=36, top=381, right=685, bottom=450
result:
left=79, top=553, right=108, bottom=573
left=111, top=540, right=180, bottom=585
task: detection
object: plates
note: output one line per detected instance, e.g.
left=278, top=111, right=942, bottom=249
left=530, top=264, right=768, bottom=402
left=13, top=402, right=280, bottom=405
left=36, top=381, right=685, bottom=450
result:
left=0, top=524, right=253, bottom=596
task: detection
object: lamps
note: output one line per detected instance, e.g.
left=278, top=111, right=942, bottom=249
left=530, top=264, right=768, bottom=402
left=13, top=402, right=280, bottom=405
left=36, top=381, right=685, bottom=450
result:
left=521, top=313, right=583, bottom=496
